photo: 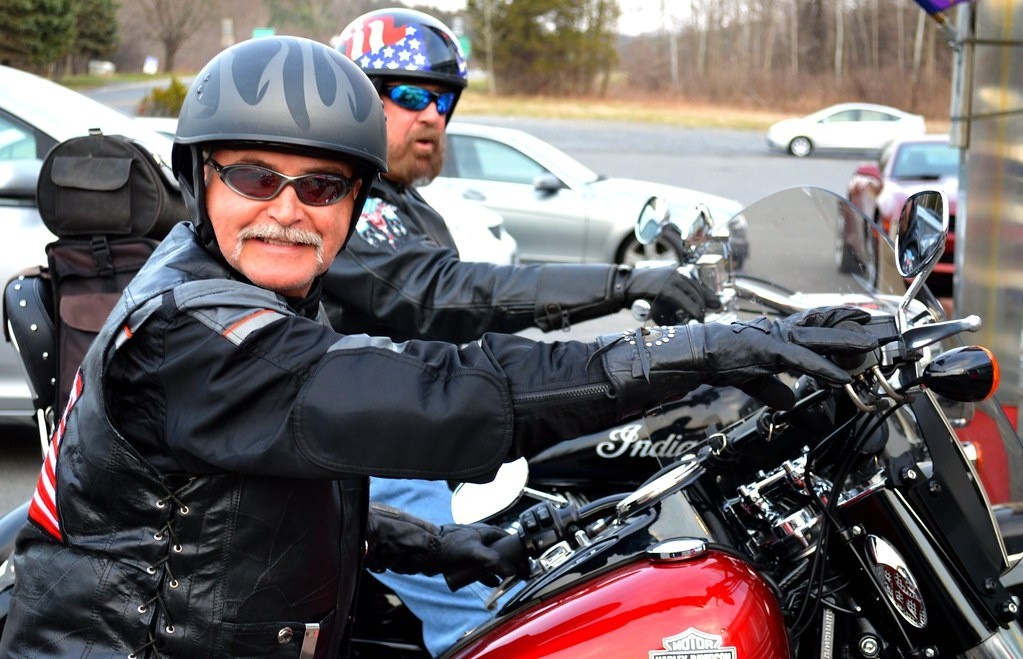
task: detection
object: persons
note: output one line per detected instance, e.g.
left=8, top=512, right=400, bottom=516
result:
left=308, top=8, right=723, bottom=650
left=0, top=34, right=882, bottom=659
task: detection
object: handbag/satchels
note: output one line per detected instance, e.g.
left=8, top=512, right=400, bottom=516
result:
left=34, top=127, right=192, bottom=243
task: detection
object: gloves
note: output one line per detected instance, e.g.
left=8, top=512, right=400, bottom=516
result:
left=362, top=503, right=532, bottom=587
left=532, top=262, right=721, bottom=324
left=595, top=305, right=879, bottom=413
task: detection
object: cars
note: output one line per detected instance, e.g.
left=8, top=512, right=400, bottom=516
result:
left=834, top=135, right=1023, bottom=289
left=414, top=123, right=752, bottom=291
left=0, top=61, right=520, bottom=421
left=765, top=102, right=927, bottom=158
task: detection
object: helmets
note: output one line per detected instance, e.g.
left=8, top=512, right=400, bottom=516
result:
left=333, top=6, right=469, bottom=131
left=170, top=35, right=389, bottom=183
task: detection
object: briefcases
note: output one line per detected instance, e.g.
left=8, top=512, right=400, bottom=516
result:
left=44, top=239, right=164, bottom=423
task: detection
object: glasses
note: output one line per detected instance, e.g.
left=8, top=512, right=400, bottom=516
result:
left=202, top=156, right=361, bottom=208
left=374, top=83, right=457, bottom=115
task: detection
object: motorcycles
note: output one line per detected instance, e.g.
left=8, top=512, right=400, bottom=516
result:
left=434, top=187, right=1023, bottom=657
